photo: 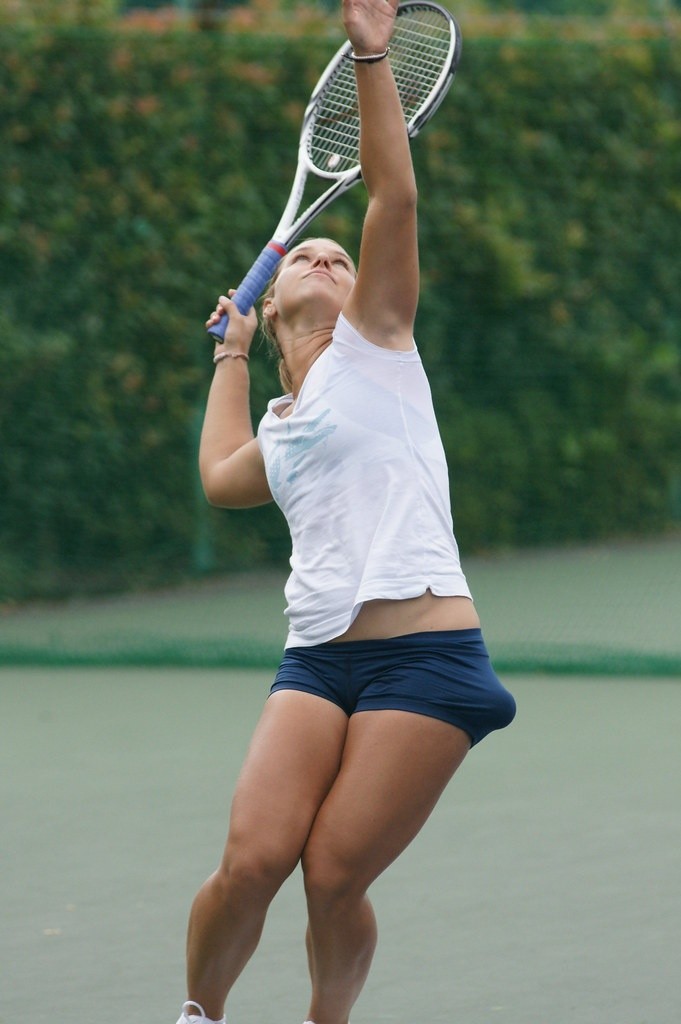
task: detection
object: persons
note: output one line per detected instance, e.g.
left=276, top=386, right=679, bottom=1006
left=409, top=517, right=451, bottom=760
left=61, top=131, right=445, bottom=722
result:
left=174, top=0, right=519, bottom=1024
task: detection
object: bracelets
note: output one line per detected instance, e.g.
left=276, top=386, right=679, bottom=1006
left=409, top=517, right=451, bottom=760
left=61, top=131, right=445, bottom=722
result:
left=342, top=43, right=392, bottom=63
left=209, top=351, right=252, bottom=365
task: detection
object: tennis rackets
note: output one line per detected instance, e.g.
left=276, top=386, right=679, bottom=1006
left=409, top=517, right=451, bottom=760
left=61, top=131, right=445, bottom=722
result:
left=206, top=2, right=465, bottom=345
